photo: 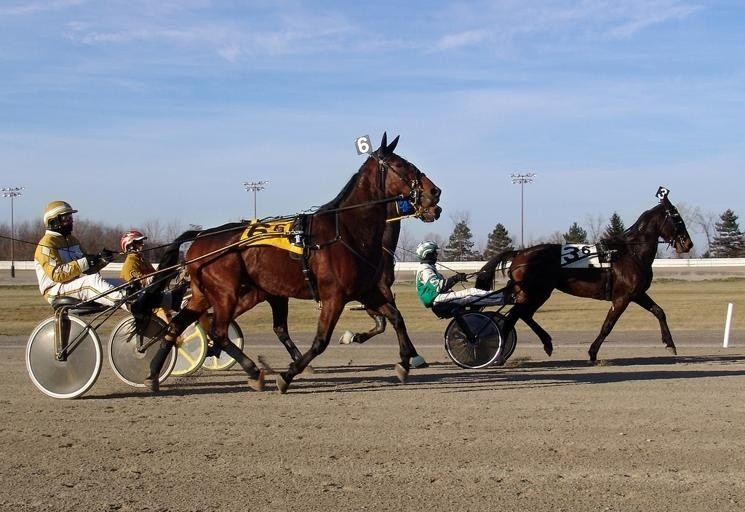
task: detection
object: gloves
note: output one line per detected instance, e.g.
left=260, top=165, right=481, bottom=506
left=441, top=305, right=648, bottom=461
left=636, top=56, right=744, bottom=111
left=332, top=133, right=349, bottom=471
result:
left=452, top=273, right=469, bottom=283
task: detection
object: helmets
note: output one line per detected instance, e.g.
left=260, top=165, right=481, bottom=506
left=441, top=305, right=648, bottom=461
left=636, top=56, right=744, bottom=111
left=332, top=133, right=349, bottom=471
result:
left=42, top=200, right=78, bottom=230
left=120, top=231, right=147, bottom=254
left=415, top=240, right=440, bottom=263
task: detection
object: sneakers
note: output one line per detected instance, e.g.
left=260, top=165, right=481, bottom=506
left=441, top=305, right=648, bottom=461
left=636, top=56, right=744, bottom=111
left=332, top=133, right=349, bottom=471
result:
left=170, top=271, right=190, bottom=313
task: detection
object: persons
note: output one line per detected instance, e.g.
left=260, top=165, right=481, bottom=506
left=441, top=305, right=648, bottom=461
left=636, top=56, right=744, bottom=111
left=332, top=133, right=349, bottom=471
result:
left=414, top=238, right=518, bottom=309
left=119, top=229, right=157, bottom=291
left=31, top=198, right=188, bottom=312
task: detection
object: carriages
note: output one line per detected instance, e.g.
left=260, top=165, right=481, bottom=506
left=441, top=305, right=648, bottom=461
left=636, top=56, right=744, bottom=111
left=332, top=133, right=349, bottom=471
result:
left=23, top=129, right=447, bottom=401
left=150, top=190, right=445, bottom=378
left=441, top=191, right=698, bottom=374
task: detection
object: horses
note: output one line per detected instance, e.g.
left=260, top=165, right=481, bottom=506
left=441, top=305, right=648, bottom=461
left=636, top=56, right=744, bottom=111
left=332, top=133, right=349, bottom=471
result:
left=470, top=194, right=694, bottom=366
left=210, top=200, right=442, bottom=376
left=120, top=131, right=442, bottom=394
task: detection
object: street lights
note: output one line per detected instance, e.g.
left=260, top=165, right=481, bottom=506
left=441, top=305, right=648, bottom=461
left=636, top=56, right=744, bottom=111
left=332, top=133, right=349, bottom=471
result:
left=0, top=183, right=24, bottom=278
left=509, top=170, right=538, bottom=248
left=239, top=177, right=269, bottom=218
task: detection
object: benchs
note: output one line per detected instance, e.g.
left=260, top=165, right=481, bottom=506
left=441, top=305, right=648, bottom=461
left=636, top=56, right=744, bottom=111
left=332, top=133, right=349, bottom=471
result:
left=61, top=277, right=134, bottom=316
